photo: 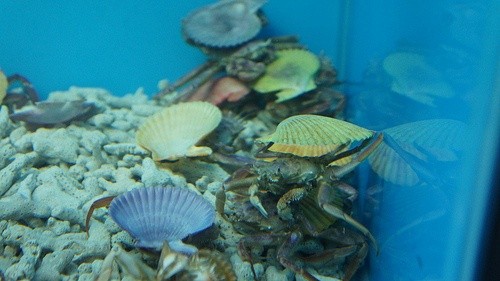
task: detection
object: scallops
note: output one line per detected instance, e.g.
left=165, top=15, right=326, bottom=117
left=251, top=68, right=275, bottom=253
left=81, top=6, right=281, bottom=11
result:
left=110, top=187, right=216, bottom=253
left=251, top=113, right=371, bottom=165
left=178, top=0, right=265, bottom=49
left=140, top=100, right=222, bottom=163
left=253, top=48, right=322, bottom=101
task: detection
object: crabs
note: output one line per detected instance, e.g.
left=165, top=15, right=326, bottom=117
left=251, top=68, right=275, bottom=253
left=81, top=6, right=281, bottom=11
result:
left=255, top=63, right=344, bottom=122
left=153, top=33, right=299, bottom=101
left=4, top=74, right=104, bottom=128
left=211, top=132, right=385, bottom=280
left=81, top=191, right=237, bottom=281
